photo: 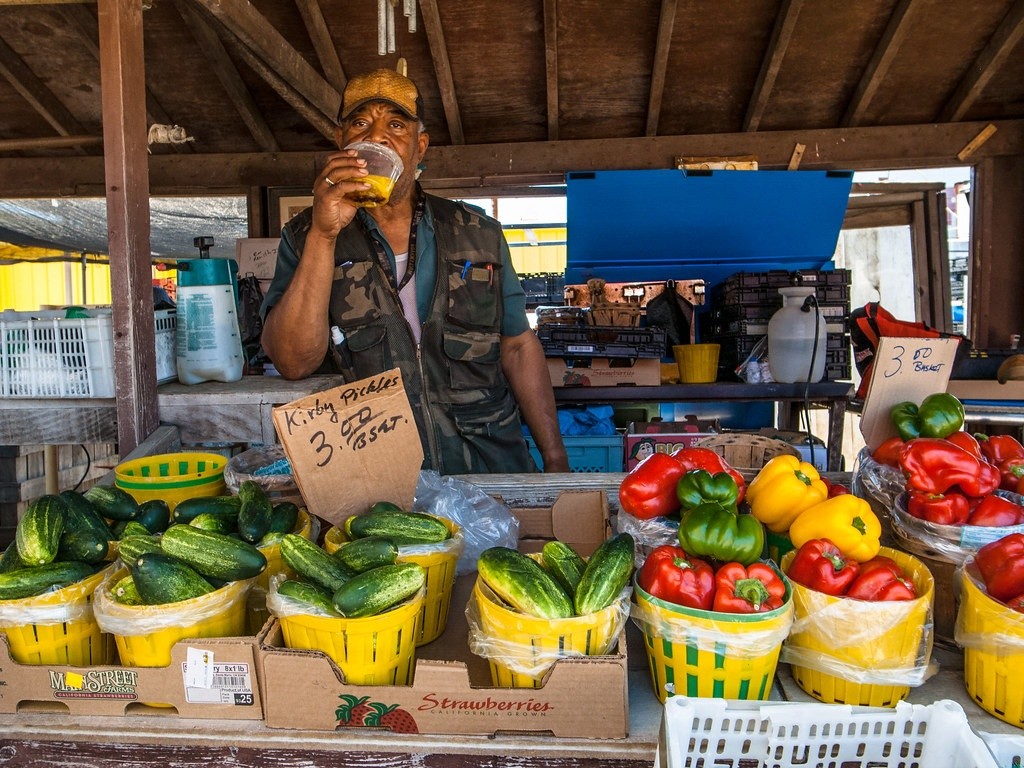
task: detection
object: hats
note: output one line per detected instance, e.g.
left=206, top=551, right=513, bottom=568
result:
left=336, top=69, right=425, bottom=128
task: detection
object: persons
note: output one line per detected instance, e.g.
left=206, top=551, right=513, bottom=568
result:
left=260, top=69, right=568, bottom=474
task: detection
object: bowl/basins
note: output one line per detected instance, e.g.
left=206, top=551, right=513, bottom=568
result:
left=673, top=344, right=720, bottom=383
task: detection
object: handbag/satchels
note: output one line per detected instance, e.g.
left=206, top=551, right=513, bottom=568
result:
left=851, top=301, right=973, bottom=399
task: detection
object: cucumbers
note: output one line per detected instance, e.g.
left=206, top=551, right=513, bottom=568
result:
left=479, top=548, right=575, bottom=621
left=543, top=542, right=588, bottom=594
left=0, top=488, right=450, bottom=618
left=575, top=532, right=633, bottom=618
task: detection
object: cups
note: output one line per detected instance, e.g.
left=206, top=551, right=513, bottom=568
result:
left=344, top=142, right=404, bottom=207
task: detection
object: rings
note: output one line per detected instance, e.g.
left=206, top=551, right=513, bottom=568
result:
left=325, top=178, right=334, bottom=186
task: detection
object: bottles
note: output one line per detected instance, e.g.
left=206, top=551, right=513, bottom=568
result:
left=768, top=287, right=827, bottom=383
left=747, top=358, right=760, bottom=384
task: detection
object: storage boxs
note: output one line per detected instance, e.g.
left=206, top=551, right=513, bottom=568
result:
left=236, top=237, right=282, bottom=291
left=0, top=307, right=181, bottom=399
left=0, top=441, right=118, bottom=550
left=0, top=491, right=628, bottom=739
left=653, top=695, right=997, bottom=768
left=712, top=268, right=852, bottom=383
left=626, top=422, right=826, bottom=474
left=538, top=326, right=669, bottom=388
left=525, top=436, right=625, bottom=473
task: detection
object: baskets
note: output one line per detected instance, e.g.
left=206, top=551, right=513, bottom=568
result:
left=654, top=695, right=1024, bottom=768
left=224, top=444, right=306, bottom=509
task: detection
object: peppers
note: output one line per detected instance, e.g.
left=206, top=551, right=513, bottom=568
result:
left=620, top=393, right=1024, bottom=614
